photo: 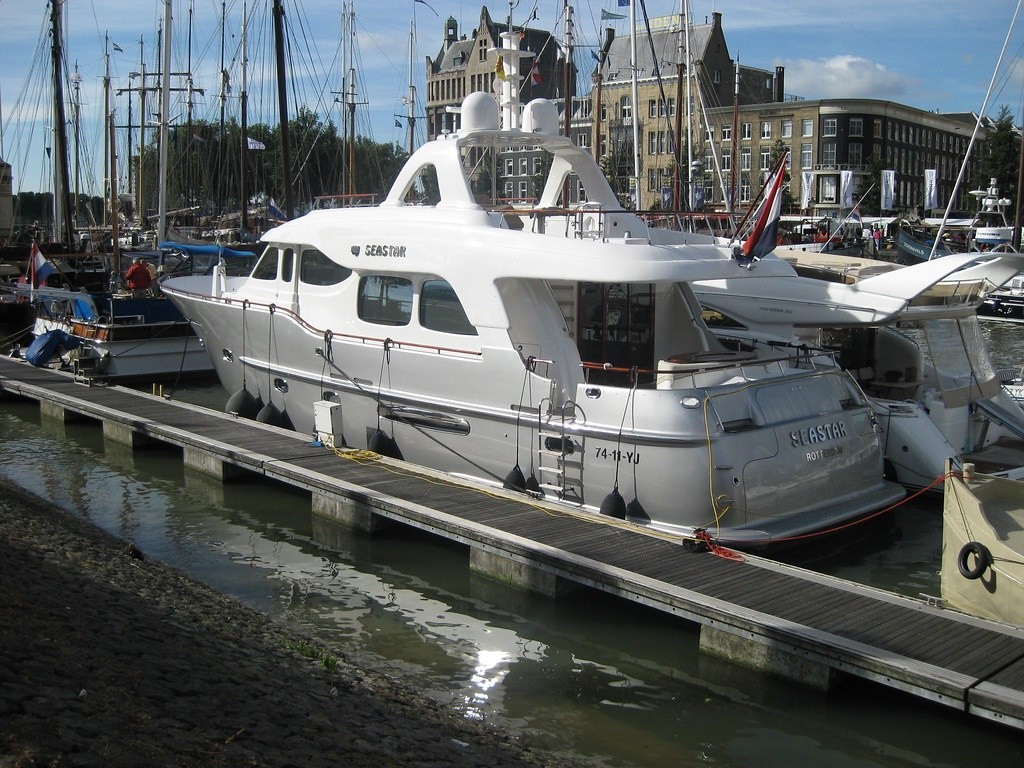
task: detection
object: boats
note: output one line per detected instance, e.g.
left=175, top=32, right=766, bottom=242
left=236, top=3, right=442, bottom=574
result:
left=151, top=1, right=910, bottom=552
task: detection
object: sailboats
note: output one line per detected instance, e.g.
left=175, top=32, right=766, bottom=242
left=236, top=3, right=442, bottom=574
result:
left=0, top=1, right=1024, bottom=637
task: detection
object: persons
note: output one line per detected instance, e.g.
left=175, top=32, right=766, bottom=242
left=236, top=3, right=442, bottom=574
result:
left=868, top=225, right=885, bottom=251
left=125, top=257, right=159, bottom=298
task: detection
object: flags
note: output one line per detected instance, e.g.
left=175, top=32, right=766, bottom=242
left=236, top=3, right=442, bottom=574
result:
left=26, top=243, right=54, bottom=309
left=601, top=8, right=627, bottom=21
left=269, top=197, right=288, bottom=221
left=247, top=136, right=265, bottom=150
left=495, top=51, right=506, bottom=81
left=531, top=59, right=543, bottom=88
left=851, top=205, right=863, bottom=229
left=743, top=151, right=789, bottom=264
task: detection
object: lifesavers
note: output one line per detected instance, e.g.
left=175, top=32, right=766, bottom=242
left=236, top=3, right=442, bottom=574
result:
left=957, top=541, right=989, bottom=579
